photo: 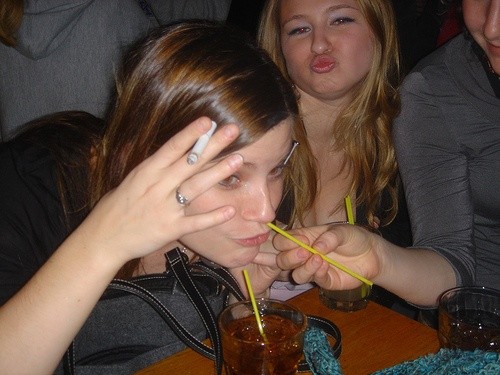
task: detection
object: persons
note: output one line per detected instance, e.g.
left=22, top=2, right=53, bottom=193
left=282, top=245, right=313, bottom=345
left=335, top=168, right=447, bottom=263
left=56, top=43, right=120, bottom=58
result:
left=272, top=1, right=500, bottom=331
left=261, top=0, right=414, bottom=307
left=0, top=0, right=298, bottom=375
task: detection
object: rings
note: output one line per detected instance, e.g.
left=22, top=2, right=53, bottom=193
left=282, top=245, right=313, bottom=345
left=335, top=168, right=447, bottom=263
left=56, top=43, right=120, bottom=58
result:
left=176, top=188, right=193, bottom=208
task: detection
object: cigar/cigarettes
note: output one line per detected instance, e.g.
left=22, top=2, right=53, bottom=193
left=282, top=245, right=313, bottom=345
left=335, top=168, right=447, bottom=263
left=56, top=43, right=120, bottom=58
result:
left=187, top=121, right=217, bottom=165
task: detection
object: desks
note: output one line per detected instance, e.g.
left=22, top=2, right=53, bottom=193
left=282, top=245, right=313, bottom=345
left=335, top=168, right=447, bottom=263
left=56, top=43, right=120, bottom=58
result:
left=131, top=285, right=441, bottom=375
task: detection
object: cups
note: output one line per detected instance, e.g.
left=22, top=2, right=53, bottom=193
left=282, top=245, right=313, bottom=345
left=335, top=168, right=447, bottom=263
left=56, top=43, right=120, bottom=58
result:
left=438, top=285, right=500, bottom=352
left=218, top=297, right=308, bottom=375
left=320, top=221, right=372, bottom=313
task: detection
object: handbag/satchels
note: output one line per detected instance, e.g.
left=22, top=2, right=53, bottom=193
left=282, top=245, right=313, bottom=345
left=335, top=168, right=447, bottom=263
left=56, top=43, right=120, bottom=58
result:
left=52, top=247, right=342, bottom=375
